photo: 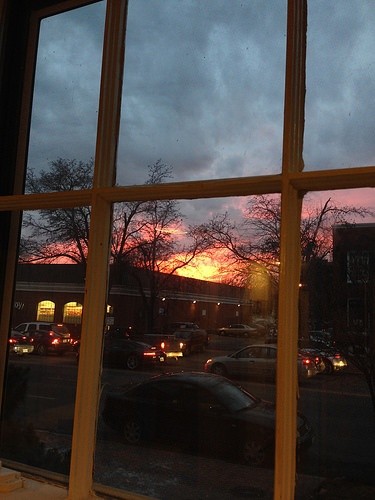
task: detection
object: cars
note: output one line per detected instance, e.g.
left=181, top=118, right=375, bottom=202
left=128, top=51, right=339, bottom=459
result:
left=129, top=323, right=211, bottom=356
left=216, top=322, right=257, bottom=338
left=8, top=322, right=82, bottom=358
left=76, top=337, right=168, bottom=371
left=204, top=344, right=320, bottom=385
left=256, top=315, right=336, bottom=350
left=266, top=337, right=348, bottom=375
left=98, top=370, right=315, bottom=464
